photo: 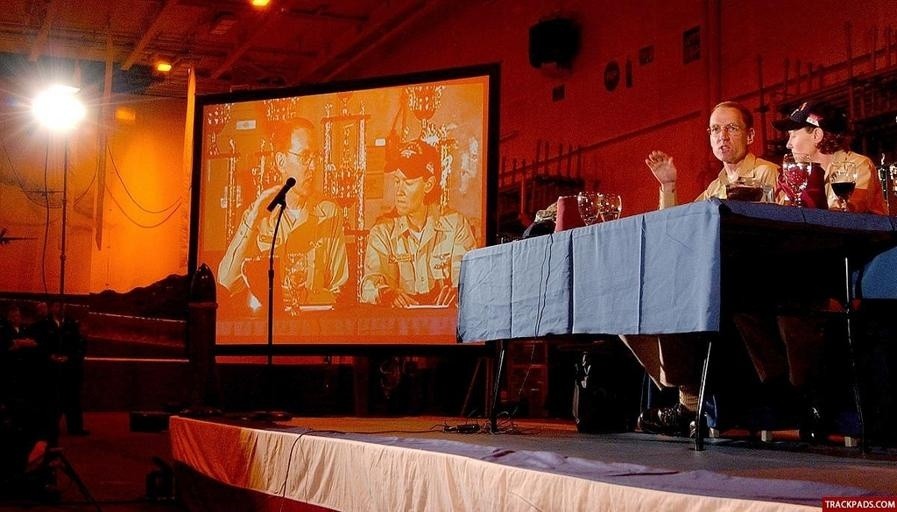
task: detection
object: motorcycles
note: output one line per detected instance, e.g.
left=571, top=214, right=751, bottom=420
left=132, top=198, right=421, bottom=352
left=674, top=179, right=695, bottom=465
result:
left=11, top=139, right=98, bottom=507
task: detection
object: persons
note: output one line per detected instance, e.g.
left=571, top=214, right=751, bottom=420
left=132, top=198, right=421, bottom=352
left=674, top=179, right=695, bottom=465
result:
left=635, top=100, right=784, bottom=439
left=728, top=98, right=889, bottom=430
left=0, top=301, right=91, bottom=437
left=360, top=138, right=478, bottom=310
left=216, top=116, right=350, bottom=305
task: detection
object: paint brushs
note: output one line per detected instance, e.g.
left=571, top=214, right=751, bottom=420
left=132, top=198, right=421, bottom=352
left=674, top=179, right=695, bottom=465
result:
left=444, top=423, right=479, bottom=433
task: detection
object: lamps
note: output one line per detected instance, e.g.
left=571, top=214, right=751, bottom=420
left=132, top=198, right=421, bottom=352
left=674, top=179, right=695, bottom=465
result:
left=759, top=270, right=863, bottom=447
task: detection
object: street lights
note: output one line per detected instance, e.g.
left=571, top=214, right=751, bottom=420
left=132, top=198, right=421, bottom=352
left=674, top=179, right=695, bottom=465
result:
left=573, top=341, right=644, bottom=433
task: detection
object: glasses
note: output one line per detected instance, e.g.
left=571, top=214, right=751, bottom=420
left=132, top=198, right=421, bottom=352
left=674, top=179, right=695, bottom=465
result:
left=48, top=82, right=80, bottom=317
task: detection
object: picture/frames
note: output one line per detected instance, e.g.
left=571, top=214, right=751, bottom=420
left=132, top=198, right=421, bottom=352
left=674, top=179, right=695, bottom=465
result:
left=456, top=198, right=897, bottom=451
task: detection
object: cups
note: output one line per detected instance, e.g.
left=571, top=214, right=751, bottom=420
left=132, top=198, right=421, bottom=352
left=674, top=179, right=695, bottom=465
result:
left=286, top=150, right=324, bottom=167
left=707, top=123, right=741, bottom=134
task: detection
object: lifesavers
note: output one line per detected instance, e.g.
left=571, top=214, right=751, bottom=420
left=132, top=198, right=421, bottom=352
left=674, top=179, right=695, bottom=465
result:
left=573, top=338, right=647, bottom=433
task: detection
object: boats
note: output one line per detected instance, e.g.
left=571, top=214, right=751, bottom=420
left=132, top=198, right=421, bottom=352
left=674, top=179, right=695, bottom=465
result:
left=526, top=15, right=584, bottom=70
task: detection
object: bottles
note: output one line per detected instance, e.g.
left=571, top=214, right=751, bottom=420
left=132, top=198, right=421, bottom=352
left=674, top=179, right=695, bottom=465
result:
left=771, top=102, right=847, bottom=133
left=385, top=141, right=441, bottom=179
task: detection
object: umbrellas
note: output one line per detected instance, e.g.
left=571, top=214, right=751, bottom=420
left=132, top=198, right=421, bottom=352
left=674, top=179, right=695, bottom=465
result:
left=638, top=402, right=709, bottom=438
left=804, top=396, right=825, bottom=419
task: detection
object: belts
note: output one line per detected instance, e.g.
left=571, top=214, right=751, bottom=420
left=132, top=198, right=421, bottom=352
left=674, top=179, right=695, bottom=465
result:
left=784, top=153, right=857, bottom=212
left=578, top=192, right=622, bottom=226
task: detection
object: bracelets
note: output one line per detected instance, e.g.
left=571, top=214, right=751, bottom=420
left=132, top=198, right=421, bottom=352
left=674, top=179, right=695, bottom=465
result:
left=726, top=178, right=764, bottom=203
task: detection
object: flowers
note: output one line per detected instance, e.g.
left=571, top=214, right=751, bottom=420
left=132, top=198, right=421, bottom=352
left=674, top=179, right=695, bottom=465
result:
left=267, top=177, right=295, bottom=211
